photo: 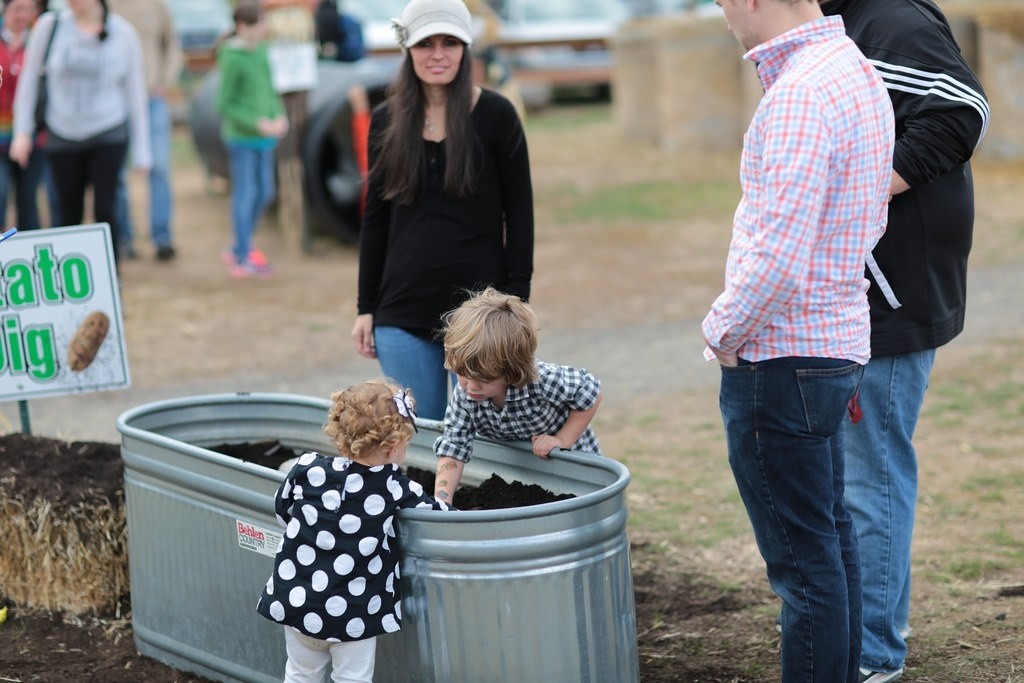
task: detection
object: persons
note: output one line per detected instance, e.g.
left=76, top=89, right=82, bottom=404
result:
left=433, top=287, right=604, bottom=506
left=701, top=0, right=895, bottom=683
left=819, top=0, right=990, bottom=683
left=352, top=0, right=534, bottom=421
left=0, top=0, right=181, bottom=263
left=214, top=6, right=289, bottom=280
left=256, top=378, right=461, bottom=683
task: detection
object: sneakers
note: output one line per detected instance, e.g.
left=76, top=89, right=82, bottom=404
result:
left=856, top=664, right=904, bottom=682
left=222, top=247, right=275, bottom=279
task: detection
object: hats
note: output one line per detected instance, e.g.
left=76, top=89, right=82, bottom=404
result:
left=390, top=0, right=474, bottom=55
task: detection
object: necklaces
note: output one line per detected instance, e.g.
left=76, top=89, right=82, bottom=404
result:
left=425, top=113, right=445, bottom=132
